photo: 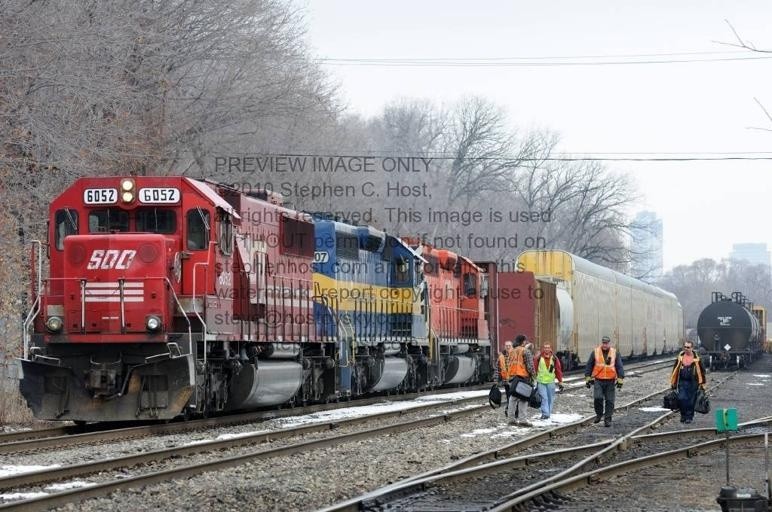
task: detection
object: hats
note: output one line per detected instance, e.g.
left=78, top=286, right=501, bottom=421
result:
left=602, top=335, right=610, bottom=344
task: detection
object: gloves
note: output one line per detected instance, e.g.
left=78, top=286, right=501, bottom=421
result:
left=557, top=384, right=566, bottom=394
left=584, top=376, right=594, bottom=389
left=614, top=377, right=624, bottom=390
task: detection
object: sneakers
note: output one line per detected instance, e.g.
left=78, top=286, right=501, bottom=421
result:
left=541, top=414, right=549, bottom=419
left=506, top=420, right=533, bottom=428
left=604, top=421, right=611, bottom=427
left=679, top=417, right=693, bottom=423
left=594, top=416, right=601, bottom=424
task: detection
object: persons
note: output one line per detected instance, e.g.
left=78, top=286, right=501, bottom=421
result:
left=582, top=334, right=626, bottom=428
left=670, top=339, right=708, bottom=424
left=487, top=333, right=565, bottom=427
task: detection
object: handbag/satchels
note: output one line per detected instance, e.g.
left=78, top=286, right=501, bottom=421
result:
left=489, top=382, right=502, bottom=404
left=694, top=385, right=712, bottom=415
left=528, top=387, right=543, bottom=408
left=509, top=377, right=533, bottom=400
left=661, top=386, right=680, bottom=411
